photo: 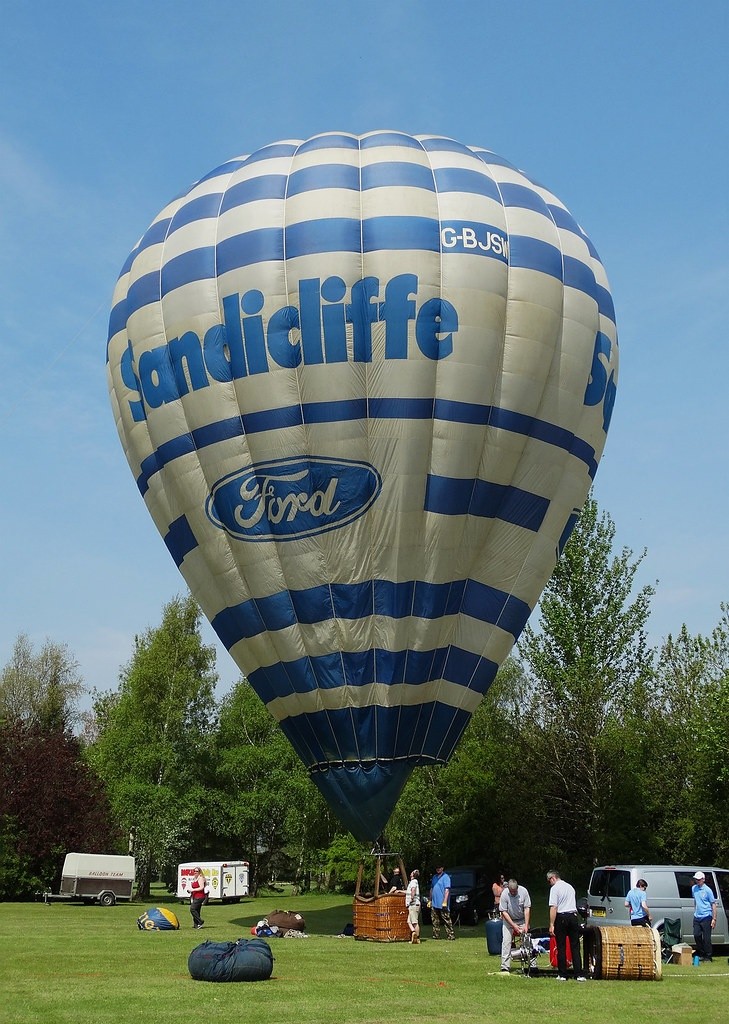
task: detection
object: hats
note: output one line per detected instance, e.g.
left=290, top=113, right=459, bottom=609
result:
left=503, top=882, right=508, bottom=886
left=693, top=871, right=705, bottom=880
left=435, top=862, right=444, bottom=868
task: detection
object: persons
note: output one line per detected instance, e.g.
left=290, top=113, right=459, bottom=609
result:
left=427, top=863, right=455, bottom=941
left=187, top=868, right=206, bottom=928
left=498, top=879, right=531, bottom=974
left=388, top=867, right=421, bottom=944
left=493, top=873, right=507, bottom=913
left=547, top=869, right=586, bottom=981
left=692, top=871, right=717, bottom=962
left=625, top=879, right=653, bottom=928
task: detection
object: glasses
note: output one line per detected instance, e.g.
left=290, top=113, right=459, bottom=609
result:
left=644, top=886, right=646, bottom=890
left=501, top=877, right=504, bottom=880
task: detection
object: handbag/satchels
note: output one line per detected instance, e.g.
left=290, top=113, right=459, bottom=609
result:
left=343, top=923, right=354, bottom=936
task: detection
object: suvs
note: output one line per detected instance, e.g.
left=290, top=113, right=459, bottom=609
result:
left=422, top=866, right=495, bottom=926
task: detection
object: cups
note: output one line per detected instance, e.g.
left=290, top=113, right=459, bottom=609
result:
left=693, top=956, right=699, bottom=965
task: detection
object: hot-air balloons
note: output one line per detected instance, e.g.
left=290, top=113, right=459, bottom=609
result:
left=102, top=128, right=621, bottom=944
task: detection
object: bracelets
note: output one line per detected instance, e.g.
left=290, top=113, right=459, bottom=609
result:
left=711, top=918, right=716, bottom=921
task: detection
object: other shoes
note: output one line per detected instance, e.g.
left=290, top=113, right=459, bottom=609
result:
left=411, top=932, right=417, bottom=944
left=576, top=976, right=586, bottom=982
left=530, top=968, right=539, bottom=973
left=197, top=922, right=205, bottom=929
left=408, top=938, right=420, bottom=944
left=699, top=957, right=712, bottom=962
left=555, top=976, right=566, bottom=980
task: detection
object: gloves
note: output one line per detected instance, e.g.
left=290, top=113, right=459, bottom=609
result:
left=427, top=901, right=431, bottom=908
left=442, top=902, right=447, bottom=907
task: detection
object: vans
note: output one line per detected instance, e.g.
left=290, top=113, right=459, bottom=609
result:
left=588, top=864, right=729, bottom=948
